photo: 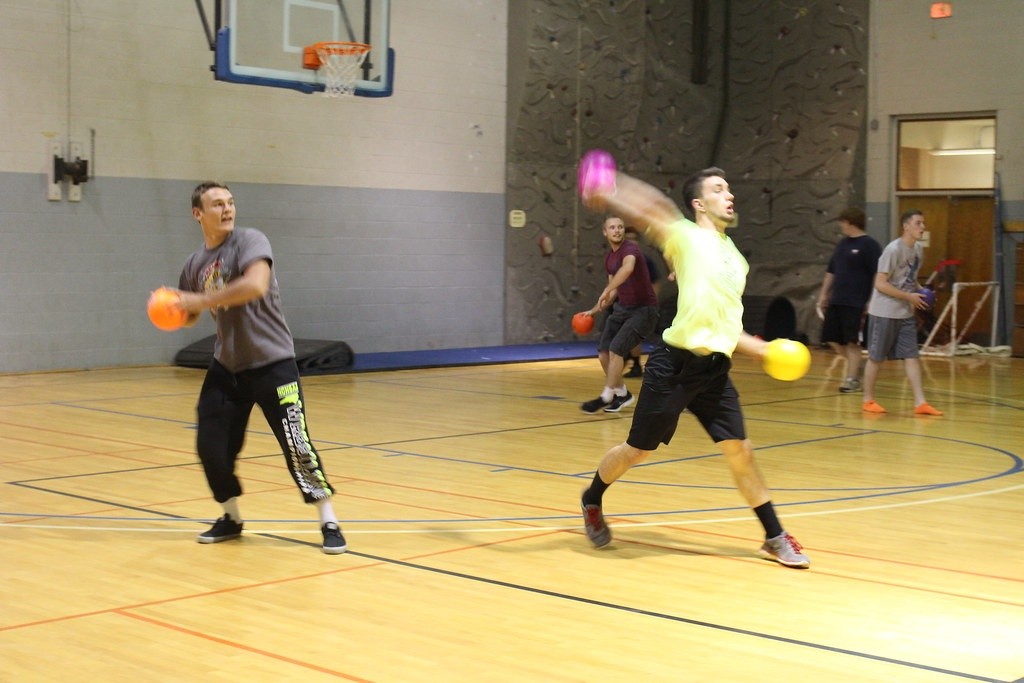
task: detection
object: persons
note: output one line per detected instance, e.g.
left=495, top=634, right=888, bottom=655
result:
left=580, top=214, right=660, bottom=415
left=162, top=180, right=348, bottom=554
left=580, top=150, right=810, bottom=569
left=815, top=206, right=883, bottom=392
left=862, top=210, right=942, bottom=416
left=623, top=227, right=667, bottom=378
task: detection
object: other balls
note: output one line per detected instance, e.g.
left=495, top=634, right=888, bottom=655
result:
left=579, top=152, right=617, bottom=193
left=918, top=288, right=935, bottom=307
left=572, top=313, right=595, bottom=335
left=764, top=338, right=811, bottom=383
left=145, top=287, right=188, bottom=334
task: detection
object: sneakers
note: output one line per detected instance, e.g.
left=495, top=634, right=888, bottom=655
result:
left=197, top=512, right=243, bottom=543
left=580, top=491, right=611, bottom=545
left=581, top=394, right=616, bottom=413
left=760, top=529, right=809, bottom=567
left=321, top=522, right=346, bottom=554
left=603, top=390, right=634, bottom=412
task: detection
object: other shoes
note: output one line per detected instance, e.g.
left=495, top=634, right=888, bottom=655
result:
left=839, top=375, right=863, bottom=392
left=622, top=365, right=643, bottom=376
